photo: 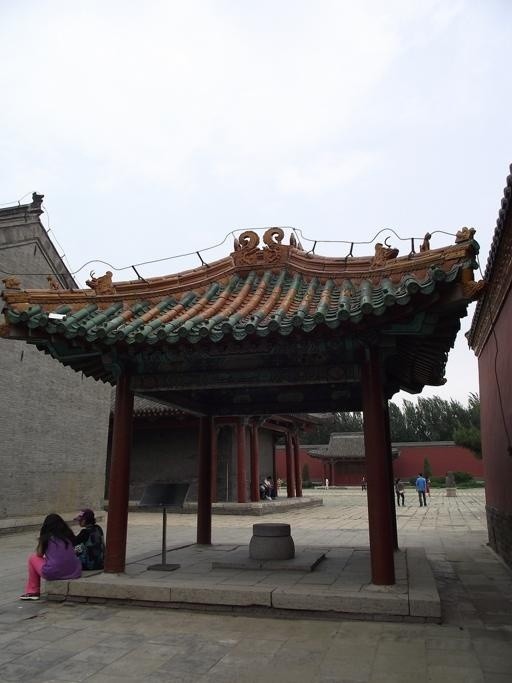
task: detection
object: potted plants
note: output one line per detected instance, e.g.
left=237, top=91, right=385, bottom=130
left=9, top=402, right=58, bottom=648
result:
left=301, top=462, right=313, bottom=489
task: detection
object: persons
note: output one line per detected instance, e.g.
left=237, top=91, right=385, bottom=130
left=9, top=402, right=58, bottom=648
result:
left=67, top=508, right=106, bottom=570
left=17, top=512, right=82, bottom=600
left=395, top=478, right=406, bottom=507
left=324, top=477, right=329, bottom=490
left=416, top=472, right=428, bottom=506
left=262, top=475, right=274, bottom=499
left=425, top=475, right=433, bottom=497
left=361, top=476, right=367, bottom=491
left=260, top=484, right=272, bottom=500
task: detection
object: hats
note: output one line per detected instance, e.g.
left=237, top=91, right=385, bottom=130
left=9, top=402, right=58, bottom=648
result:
left=72, top=508, right=94, bottom=520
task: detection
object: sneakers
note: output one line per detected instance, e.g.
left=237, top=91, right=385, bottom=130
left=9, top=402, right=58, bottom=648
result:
left=20, top=593, right=40, bottom=600
